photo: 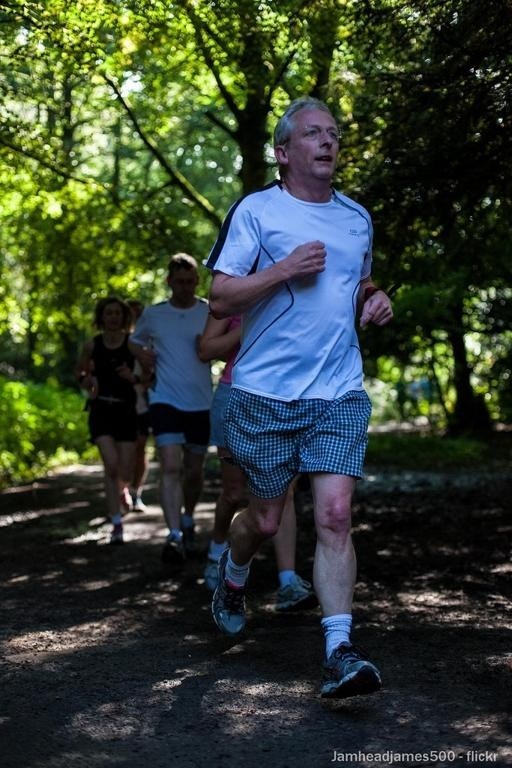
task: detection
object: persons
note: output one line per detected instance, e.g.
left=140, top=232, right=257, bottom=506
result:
left=210, top=93, right=394, bottom=702
left=76, top=296, right=148, bottom=540
left=198, top=306, right=312, bottom=614
left=129, top=253, right=212, bottom=559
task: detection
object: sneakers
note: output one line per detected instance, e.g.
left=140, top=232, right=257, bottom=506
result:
left=117, top=494, right=144, bottom=516
left=204, top=539, right=230, bottom=592
left=167, top=514, right=196, bottom=542
left=111, top=522, right=123, bottom=545
left=275, top=579, right=319, bottom=611
left=319, top=642, right=382, bottom=698
left=211, top=547, right=251, bottom=637
